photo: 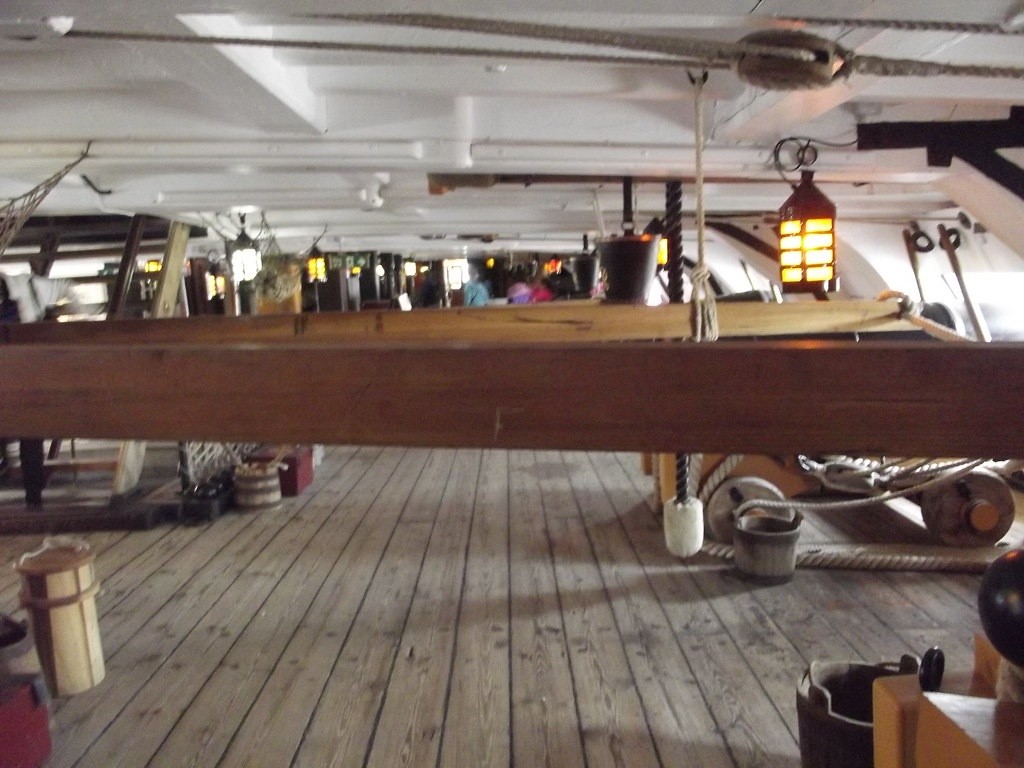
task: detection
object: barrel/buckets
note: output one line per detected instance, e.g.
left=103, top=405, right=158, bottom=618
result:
left=795, top=653, right=922, bottom=768
left=728, top=509, right=804, bottom=580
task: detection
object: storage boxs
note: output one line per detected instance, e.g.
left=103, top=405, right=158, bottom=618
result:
left=874, top=631, right=1024, bottom=768
left=245, top=444, right=313, bottom=497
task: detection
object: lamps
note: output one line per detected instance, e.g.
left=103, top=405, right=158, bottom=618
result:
left=306, top=238, right=327, bottom=285
left=145, top=260, right=162, bottom=272
left=779, top=169, right=843, bottom=294
left=643, top=217, right=668, bottom=274
left=231, top=212, right=263, bottom=292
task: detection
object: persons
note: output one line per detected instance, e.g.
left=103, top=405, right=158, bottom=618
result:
left=463, top=266, right=489, bottom=307
left=507, top=278, right=553, bottom=304
